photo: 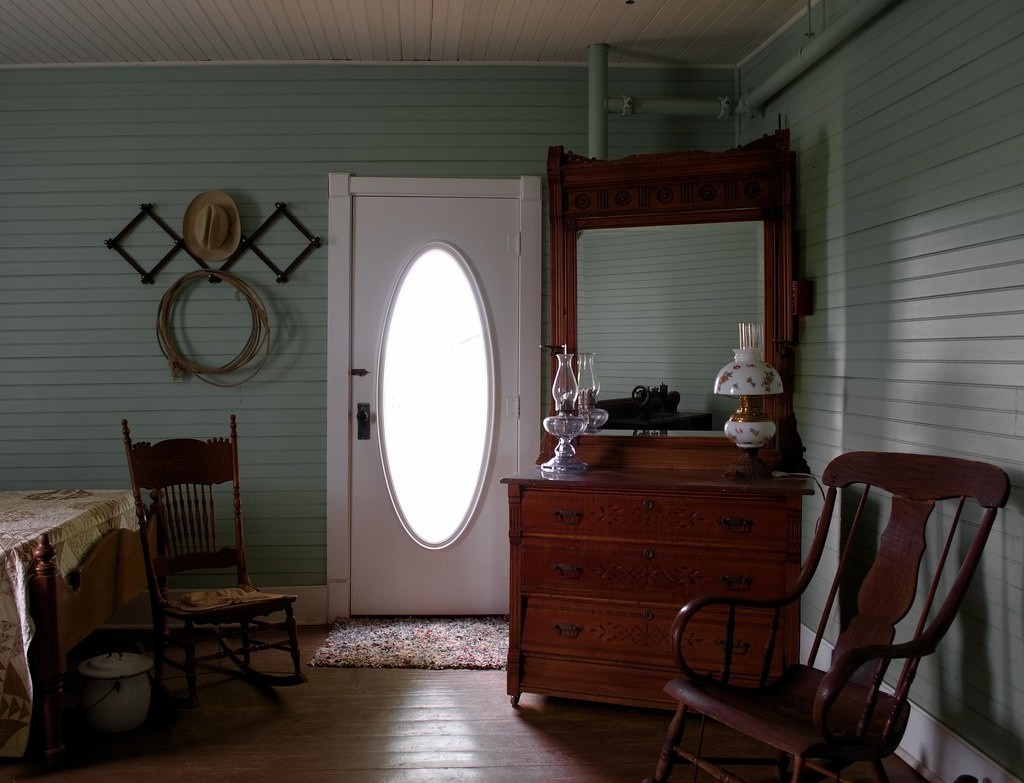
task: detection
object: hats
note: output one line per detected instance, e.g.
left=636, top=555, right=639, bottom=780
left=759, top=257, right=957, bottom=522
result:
left=182, top=190, right=242, bottom=263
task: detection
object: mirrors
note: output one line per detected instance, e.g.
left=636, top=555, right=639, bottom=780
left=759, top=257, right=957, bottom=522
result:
left=547, top=129, right=800, bottom=472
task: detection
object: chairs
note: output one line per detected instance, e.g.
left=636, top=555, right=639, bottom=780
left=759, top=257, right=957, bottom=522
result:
left=122, top=415, right=308, bottom=708
left=654, top=451, right=1011, bottom=783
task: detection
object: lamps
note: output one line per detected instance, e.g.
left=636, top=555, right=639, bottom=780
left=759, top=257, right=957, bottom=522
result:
left=714, top=322, right=784, bottom=481
left=576, top=352, right=608, bottom=435
left=541, top=354, right=589, bottom=475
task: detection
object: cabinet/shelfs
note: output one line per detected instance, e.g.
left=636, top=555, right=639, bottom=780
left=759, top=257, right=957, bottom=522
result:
left=501, top=465, right=816, bottom=712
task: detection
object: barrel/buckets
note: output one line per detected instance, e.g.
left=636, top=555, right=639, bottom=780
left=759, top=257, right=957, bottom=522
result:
left=79, top=651, right=154, bottom=733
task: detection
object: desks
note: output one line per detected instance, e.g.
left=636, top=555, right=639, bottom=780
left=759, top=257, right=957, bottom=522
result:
left=0, top=490, right=171, bottom=753
